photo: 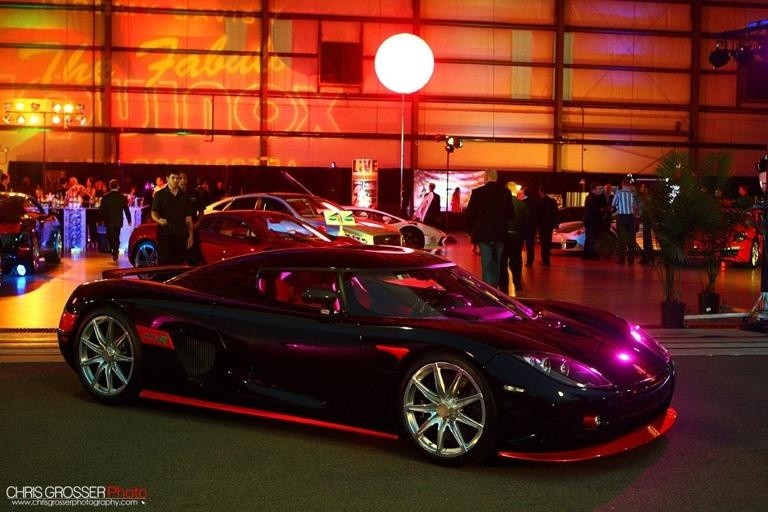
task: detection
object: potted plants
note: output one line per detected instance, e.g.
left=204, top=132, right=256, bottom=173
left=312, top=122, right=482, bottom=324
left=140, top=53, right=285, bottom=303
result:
left=628, top=143, right=749, bottom=325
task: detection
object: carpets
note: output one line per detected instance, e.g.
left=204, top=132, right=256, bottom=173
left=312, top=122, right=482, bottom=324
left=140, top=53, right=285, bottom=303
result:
left=0, top=355, right=768, bottom=512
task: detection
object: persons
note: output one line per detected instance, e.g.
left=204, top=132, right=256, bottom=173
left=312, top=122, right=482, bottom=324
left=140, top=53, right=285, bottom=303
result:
left=1, top=172, right=227, bottom=281
left=424, top=182, right=441, bottom=230
left=450, top=186, right=462, bottom=213
left=466, top=167, right=559, bottom=295
left=580, top=178, right=656, bottom=266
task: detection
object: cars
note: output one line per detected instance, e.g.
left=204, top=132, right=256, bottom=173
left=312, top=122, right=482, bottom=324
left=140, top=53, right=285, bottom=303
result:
left=205, top=191, right=401, bottom=245
left=553, top=213, right=663, bottom=253
left=128, top=210, right=368, bottom=282
left=0, top=190, right=63, bottom=289
left=57, top=247, right=679, bottom=469
left=341, top=205, right=448, bottom=255
left=684, top=208, right=764, bottom=268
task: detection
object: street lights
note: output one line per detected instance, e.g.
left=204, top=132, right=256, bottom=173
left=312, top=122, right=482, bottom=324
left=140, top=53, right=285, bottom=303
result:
left=749, top=157, right=767, bottom=320
